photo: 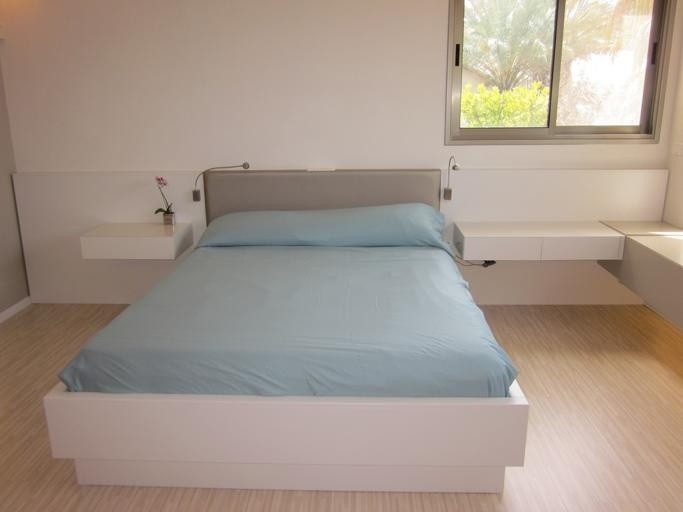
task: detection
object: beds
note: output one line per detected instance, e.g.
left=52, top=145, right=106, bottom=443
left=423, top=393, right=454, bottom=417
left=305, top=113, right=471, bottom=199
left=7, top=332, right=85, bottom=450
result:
left=44, top=170, right=530, bottom=492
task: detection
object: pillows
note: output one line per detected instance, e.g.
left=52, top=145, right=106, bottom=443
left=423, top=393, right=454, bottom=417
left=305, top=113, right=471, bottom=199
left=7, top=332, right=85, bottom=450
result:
left=188, top=200, right=461, bottom=261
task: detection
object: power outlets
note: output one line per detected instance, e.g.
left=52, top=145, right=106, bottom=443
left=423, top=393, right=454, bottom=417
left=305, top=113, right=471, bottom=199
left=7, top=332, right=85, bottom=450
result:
left=483, top=260, right=496, bottom=266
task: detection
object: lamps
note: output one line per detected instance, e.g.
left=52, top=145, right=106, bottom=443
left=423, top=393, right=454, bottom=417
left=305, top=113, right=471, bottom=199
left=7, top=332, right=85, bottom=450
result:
left=443, top=155, right=461, bottom=200
left=192, top=161, right=250, bottom=202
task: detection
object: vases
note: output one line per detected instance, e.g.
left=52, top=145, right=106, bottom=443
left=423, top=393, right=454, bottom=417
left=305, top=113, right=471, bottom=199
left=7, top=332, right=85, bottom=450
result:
left=162, top=215, right=175, bottom=225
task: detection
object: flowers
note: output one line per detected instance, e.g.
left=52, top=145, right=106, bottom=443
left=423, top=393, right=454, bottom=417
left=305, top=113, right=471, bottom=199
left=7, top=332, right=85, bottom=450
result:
left=154, top=176, right=173, bottom=214
